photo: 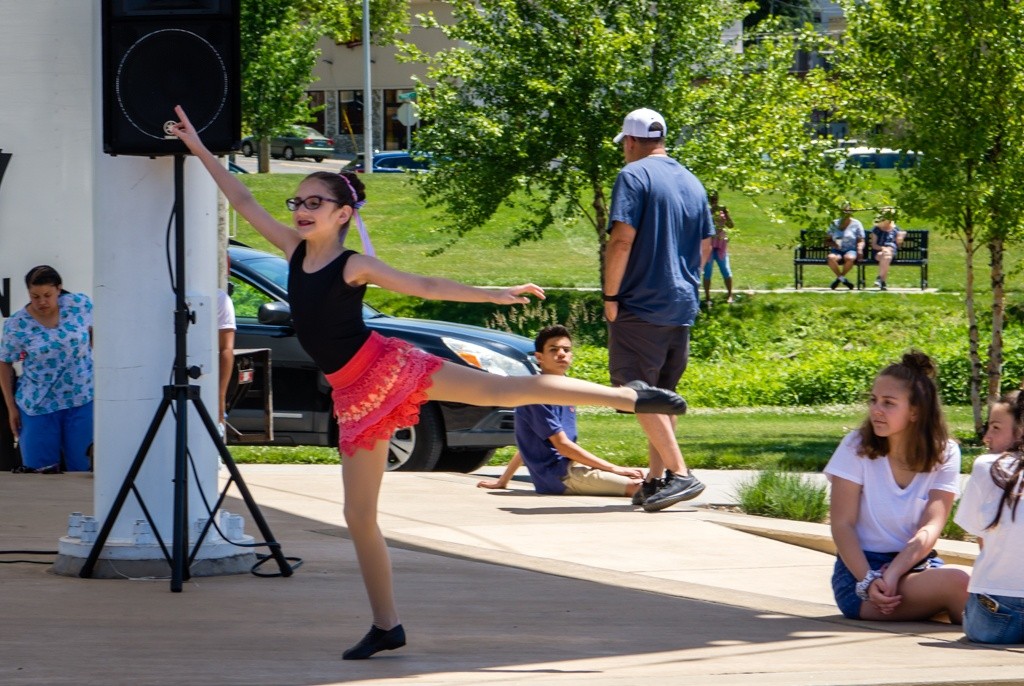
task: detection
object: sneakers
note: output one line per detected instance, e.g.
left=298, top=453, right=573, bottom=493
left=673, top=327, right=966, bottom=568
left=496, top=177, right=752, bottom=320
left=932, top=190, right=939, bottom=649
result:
left=641, top=467, right=705, bottom=511
left=631, top=476, right=664, bottom=505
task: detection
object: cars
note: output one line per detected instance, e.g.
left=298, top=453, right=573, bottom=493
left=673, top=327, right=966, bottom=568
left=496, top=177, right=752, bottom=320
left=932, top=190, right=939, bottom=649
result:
left=243, top=126, right=334, bottom=163
left=225, top=237, right=564, bottom=474
left=341, top=150, right=465, bottom=173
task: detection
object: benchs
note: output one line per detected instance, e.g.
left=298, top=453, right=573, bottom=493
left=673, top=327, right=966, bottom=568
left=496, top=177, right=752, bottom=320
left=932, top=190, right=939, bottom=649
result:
left=792, top=229, right=930, bottom=290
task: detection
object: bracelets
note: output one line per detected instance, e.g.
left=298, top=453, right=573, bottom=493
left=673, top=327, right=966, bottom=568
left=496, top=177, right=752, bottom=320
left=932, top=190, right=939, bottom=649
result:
left=855, top=569, right=883, bottom=601
left=601, top=292, right=622, bottom=302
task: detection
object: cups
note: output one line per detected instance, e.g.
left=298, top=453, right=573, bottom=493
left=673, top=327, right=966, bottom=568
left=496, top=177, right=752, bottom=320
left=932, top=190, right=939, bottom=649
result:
left=835, top=237, right=842, bottom=249
left=897, top=231, right=905, bottom=243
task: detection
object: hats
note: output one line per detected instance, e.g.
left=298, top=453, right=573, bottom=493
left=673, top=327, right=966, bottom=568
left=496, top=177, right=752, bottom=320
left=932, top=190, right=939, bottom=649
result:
left=613, top=107, right=667, bottom=144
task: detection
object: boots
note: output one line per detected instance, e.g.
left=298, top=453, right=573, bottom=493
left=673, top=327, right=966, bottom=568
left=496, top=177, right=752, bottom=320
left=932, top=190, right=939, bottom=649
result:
left=725, top=278, right=733, bottom=303
left=703, top=279, right=712, bottom=300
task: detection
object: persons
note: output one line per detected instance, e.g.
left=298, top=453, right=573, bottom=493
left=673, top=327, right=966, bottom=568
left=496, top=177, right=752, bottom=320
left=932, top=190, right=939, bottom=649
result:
left=822, top=348, right=972, bottom=626
left=601, top=107, right=717, bottom=514
left=167, top=103, right=689, bottom=661
left=216, top=283, right=238, bottom=465
left=952, top=379, right=1024, bottom=644
left=868, top=206, right=907, bottom=291
left=0, top=265, right=96, bottom=473
left=821, top=201, right=865, bottom=290
left=700, top=188, right=736, bottom=306
left=475, top=324, right=667, bottom=497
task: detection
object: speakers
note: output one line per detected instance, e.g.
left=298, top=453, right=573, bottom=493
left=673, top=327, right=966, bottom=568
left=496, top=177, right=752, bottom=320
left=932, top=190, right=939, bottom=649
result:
left=101, top=0, right=243, bottom=158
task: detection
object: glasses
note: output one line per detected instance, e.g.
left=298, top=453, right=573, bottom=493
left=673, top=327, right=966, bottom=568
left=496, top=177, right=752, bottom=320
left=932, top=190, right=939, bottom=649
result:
left=286, top=196, right=343, bottom=212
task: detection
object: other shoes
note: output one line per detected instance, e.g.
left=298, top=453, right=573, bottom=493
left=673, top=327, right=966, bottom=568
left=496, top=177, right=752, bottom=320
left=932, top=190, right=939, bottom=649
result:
left=874, top=275, right=887, bottom=291
left=831, top=278, right=841, bottom=289
left=616, top=380, right=689, bottom=417
left=842, top=279, right=854, bottom=289
left=341, top=622, right=406, bottom=659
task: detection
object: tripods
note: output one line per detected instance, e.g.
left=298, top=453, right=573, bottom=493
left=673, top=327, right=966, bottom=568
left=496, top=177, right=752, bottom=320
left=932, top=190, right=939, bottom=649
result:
left=78, top=156, right=295, bottom=594
